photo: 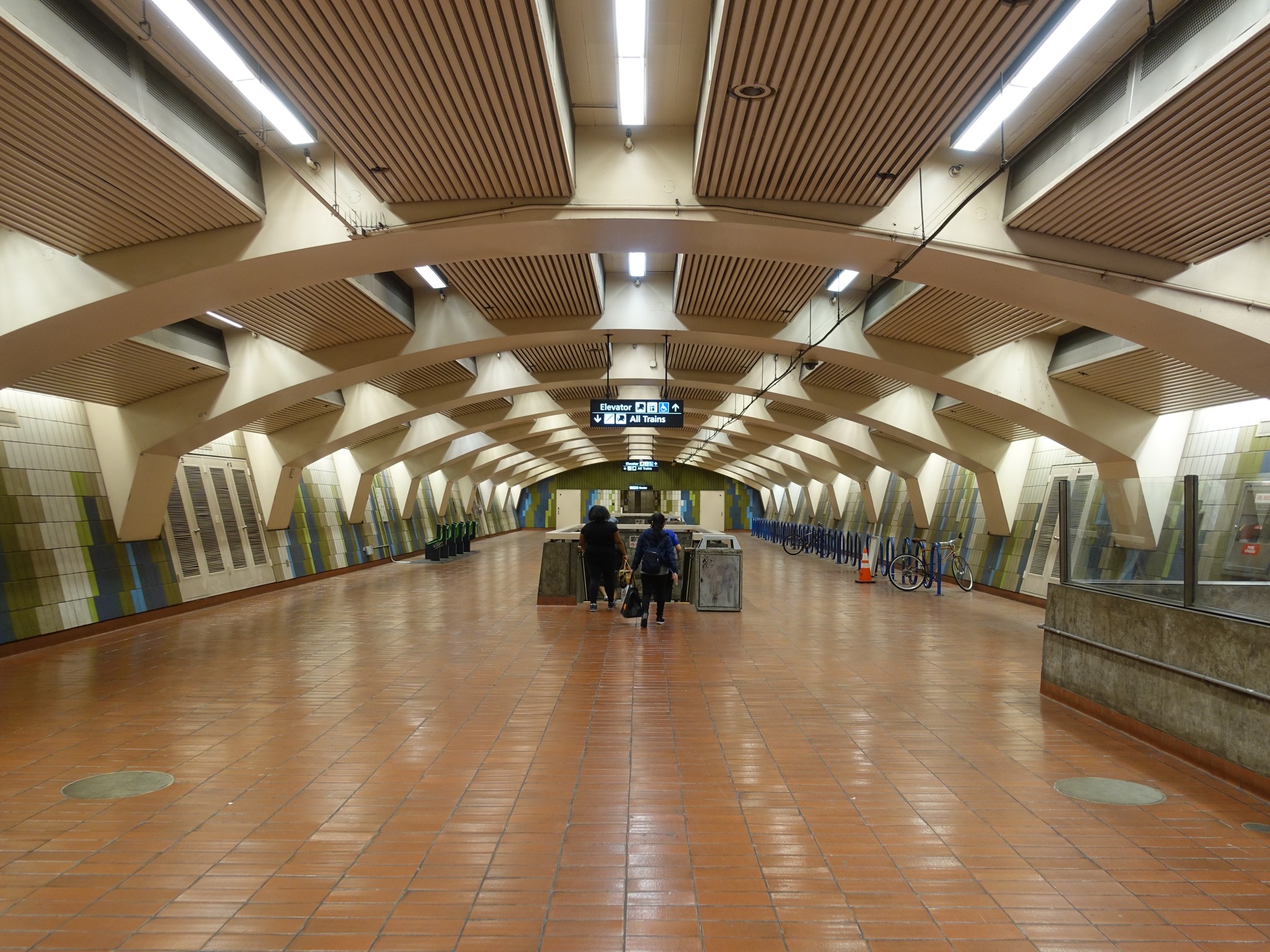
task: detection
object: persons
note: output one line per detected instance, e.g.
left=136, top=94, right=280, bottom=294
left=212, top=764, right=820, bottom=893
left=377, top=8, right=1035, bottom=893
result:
left=600, top=517, right=631, bottom=602
left=647, top=517, right=681, bottom=603
left=580, top=505, right=629, bottom=611
left=631, top=513, right=678, bottom=627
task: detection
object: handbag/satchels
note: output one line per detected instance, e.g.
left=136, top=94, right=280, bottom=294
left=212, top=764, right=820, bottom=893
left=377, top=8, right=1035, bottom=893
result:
left=621, top=584, right=633, bottom=602
left=620, top=572, right=642, bottom=618
left=618, top=559, right=635, bottom=585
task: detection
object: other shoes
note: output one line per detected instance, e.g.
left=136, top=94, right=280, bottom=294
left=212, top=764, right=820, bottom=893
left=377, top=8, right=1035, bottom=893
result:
left=604, top=596, right=608, bottom=601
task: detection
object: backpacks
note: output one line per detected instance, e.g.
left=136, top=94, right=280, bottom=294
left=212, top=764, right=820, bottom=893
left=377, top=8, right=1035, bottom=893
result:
left=641, top=532, right=669, bottom=574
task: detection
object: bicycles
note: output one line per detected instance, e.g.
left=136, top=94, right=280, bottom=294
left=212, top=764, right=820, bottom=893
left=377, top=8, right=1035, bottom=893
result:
left=783, top=521, right=837, bottom=555
left=888, top=532, right=973, bottom=592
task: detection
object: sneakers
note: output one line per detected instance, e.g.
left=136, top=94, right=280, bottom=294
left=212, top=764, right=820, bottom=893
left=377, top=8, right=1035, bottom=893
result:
left=608, top=601, right=617, bottom=609
left=590, top=603, right=598, bottom=611
left=656, top=617, right=665, bottom=624
left=640, top=612, right=648, bottom=627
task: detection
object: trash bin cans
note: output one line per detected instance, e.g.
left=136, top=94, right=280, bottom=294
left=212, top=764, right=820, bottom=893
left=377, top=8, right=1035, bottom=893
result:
left=694, top=534, right=744, bottom=612
left=666, top=516, right=685, bottom=525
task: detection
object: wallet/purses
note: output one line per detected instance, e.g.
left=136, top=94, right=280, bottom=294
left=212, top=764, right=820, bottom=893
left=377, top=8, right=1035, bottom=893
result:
left=672, top=574, right=678, bottom=585
